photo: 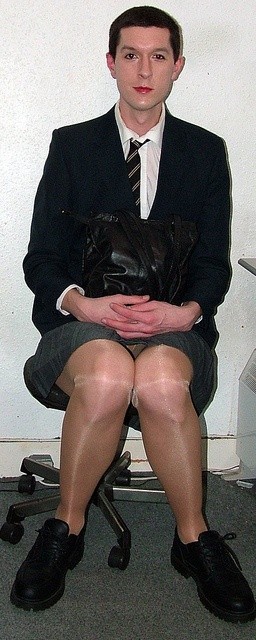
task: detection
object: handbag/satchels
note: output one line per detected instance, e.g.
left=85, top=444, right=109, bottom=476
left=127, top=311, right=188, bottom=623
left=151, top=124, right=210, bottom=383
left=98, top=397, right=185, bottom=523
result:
left=59, top=202, right=201, bottom=306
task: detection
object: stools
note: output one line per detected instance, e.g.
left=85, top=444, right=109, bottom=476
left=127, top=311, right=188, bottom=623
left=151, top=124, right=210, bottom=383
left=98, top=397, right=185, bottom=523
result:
left=0, top=354, right=137, bottom=570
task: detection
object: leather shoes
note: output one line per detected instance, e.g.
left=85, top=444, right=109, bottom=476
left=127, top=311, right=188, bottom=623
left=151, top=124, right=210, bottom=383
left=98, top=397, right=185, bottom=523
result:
left=10, top=518, right=86, bottom=612
left=170, top=526, right=256, bottom=624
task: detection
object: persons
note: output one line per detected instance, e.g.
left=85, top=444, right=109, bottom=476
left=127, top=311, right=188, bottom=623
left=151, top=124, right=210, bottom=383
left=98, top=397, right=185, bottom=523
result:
left=10, top=5, right=255, bottom=624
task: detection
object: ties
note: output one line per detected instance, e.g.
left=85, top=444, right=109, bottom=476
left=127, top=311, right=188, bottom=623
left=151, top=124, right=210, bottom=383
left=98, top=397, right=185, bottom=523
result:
left=125, top=138, right=151, bottom=219
